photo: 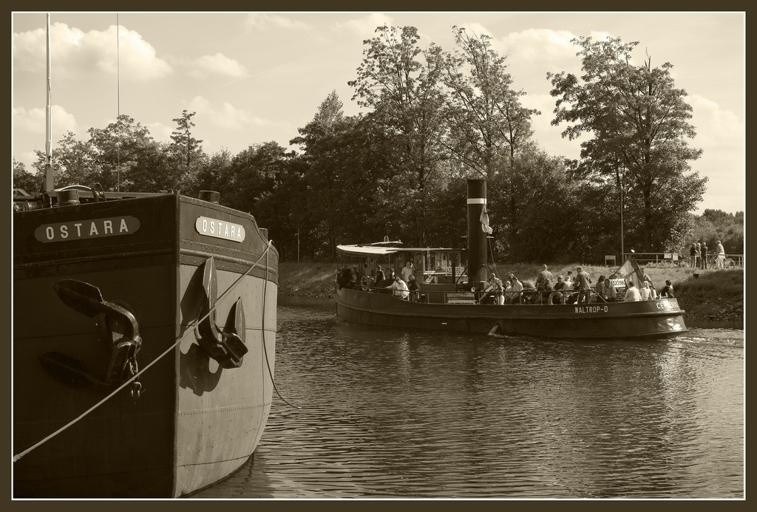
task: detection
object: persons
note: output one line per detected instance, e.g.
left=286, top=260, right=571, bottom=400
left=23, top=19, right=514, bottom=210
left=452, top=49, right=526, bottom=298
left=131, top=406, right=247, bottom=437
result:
left=700, top=241, right=707, bottom=270
left=596, top=275, right=616, bottom=302
left=533, top=265, right=593, bottom=304
left=694, top=242, right=701, bottom=268
left=476, top=272, right=535, bottom=304
left=689, top=242, right=696, bottom=270
left=712, top=240, right=724, bottom=270
left=338, top=260, right=416, bottom=300
left=626, top=280, right=673, bottom=302
